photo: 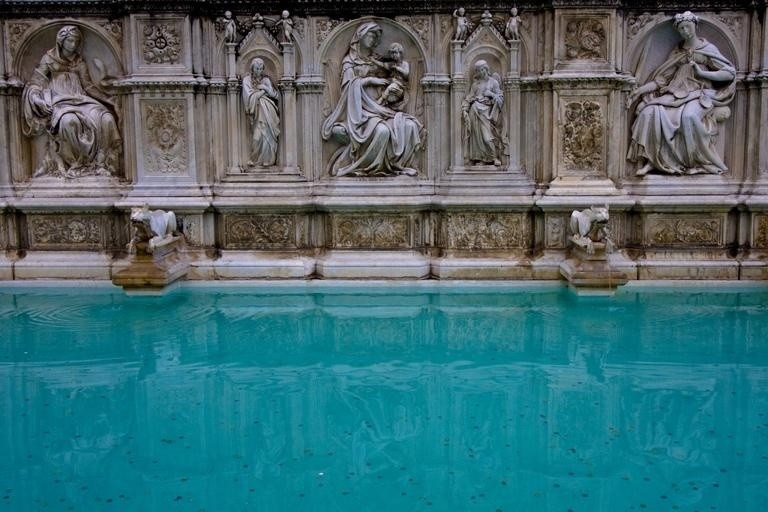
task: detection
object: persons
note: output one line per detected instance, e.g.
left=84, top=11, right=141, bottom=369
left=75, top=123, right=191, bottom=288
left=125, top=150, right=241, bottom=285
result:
left=461, top=59, right=504, bottom=166
left=19, top=25, right=123, bottom=179
left=369, top=42, right=410, bottom=105
left=453, top=7, right=468, bottom=41
left=506, top=8, right=522, bottom=39
left=222, top=10, right=236, bottom=42
left=275, top=10, right=294, bottom=44
left=321, top=22, right=424, bottom=177
left=625, top=11, right=737, bottom=177
left=242, top=57, right=280, bottom=167
